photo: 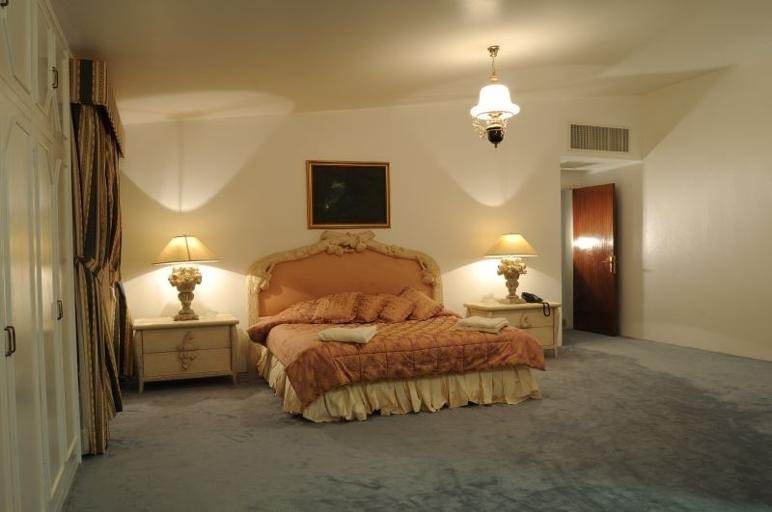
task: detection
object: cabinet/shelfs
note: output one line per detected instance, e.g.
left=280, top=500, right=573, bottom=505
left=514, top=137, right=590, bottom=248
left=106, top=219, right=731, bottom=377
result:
left=1, top=0, right=84, bottom=512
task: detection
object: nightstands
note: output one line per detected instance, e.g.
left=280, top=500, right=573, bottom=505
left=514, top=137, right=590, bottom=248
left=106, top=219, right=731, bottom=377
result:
left=462, top=301, right=562, bottom=358
left=131, top=313, right=241, bottom=394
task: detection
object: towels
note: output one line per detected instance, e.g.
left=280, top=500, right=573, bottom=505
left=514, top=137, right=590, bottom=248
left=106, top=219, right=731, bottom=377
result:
left=455, top=315, right=510, bottom=334
left=319, top=325, right=378, bottom=344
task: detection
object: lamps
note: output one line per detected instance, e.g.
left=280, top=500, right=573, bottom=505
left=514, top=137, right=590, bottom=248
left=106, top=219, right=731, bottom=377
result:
left=469, top=44, right=521, bottom=149
left=150, top=234, right=219, bottom=321
left=483, top=232, right=539, bottom=305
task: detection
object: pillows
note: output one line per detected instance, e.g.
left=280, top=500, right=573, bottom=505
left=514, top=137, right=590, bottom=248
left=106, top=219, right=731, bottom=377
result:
left=310, top=286, right=443, bottom=326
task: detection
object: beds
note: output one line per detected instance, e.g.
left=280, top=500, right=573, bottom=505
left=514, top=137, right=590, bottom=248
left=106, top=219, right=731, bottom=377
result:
left=247, top=232, right=533, bottom=424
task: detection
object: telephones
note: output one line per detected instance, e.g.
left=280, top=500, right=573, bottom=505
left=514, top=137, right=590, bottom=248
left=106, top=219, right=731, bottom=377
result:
left=522, top=292, right=541, bottom=302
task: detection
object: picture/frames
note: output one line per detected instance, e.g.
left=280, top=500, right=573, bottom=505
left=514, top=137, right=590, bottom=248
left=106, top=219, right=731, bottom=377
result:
left=305, top=159, right=392, bottom=230
